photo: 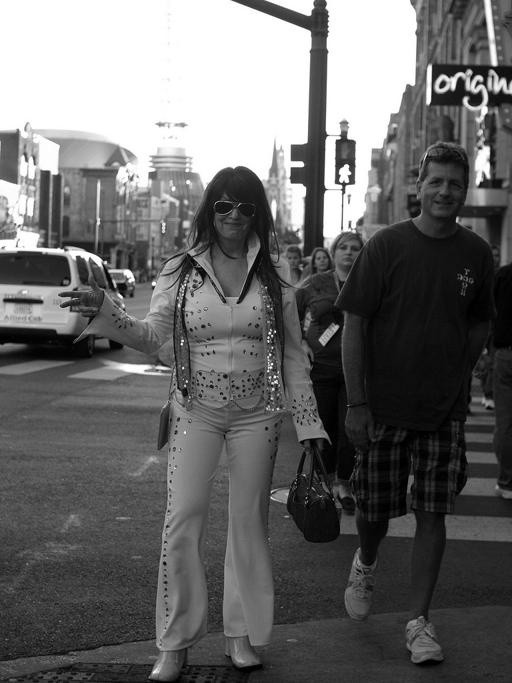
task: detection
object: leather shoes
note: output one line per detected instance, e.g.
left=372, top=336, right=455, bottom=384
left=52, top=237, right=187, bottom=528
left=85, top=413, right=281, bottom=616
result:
left=150, top=650, right=186, bottom=680
left=226, top=635, right=262, bottom=667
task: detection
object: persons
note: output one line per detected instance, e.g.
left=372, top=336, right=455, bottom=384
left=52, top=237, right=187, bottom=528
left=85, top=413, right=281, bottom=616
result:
left=58, top=165, right=333, bottom=682
left=298, top=247, right=333, bottom=338
left=334, top=140, right=499, bottom=665
left=293, top=231, right=365, bottom=510
left=286, top=244, right=302, bottom=285
left=483, top=260, right=512, bottom=500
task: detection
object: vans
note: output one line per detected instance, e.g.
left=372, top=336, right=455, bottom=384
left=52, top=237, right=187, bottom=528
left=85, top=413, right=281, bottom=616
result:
left=1, top=243, right=125, bottom=354
left=108, top=267, right=134, bottom=297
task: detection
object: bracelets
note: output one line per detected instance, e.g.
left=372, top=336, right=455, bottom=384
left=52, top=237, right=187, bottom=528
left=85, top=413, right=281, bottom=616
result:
left=346, top=401, right=369, bottom=407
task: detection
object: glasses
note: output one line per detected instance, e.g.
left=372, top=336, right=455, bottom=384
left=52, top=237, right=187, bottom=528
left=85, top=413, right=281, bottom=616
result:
left=211, top=200, right=255, bottom=219
left=423, top=148, right=466, bottom=163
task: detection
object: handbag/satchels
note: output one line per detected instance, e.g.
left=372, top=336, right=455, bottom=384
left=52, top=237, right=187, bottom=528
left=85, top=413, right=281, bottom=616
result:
left=287, top=447, right=340, bottom=542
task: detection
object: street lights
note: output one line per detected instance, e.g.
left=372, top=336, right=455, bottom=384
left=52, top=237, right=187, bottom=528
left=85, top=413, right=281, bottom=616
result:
left=336, top=116, right=351, bottom=232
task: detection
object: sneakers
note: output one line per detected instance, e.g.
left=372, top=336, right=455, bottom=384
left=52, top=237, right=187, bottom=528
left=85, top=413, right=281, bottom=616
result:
left=481, top=396, right=495, bottom=410
left=495, top=484, right=512, bottom=498
left=345, top=548, right=379, bottom=621
left=336, top=489, right=356, bottom=512
left=404, top=617, right=444, bottom=664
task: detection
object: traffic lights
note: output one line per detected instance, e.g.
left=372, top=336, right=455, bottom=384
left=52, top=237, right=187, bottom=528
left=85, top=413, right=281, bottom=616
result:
left=335, top=139, right=356, bottom=185
left=161, top=222, right=166, bottom=234
left=290, top=139, right=310, bottom=189
